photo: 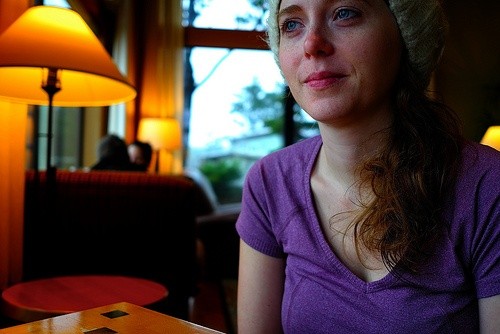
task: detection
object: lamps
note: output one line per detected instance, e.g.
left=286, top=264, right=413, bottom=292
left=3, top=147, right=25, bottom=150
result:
left=0, top=5, right=138, bottom=169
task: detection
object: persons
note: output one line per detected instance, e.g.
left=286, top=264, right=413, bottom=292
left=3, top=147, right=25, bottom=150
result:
left=89, top=134, right=154, bottom=172
left=235, top=0, right=499, bottom=334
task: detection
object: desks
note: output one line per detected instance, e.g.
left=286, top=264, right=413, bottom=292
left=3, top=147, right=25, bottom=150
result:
left=0, top=302, right=227, bottom=334
left=1, top=274, right=169, bottom=324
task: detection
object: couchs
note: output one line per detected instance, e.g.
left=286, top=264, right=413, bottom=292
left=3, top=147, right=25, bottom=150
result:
left=22, top=168, right=244, bottom=319
